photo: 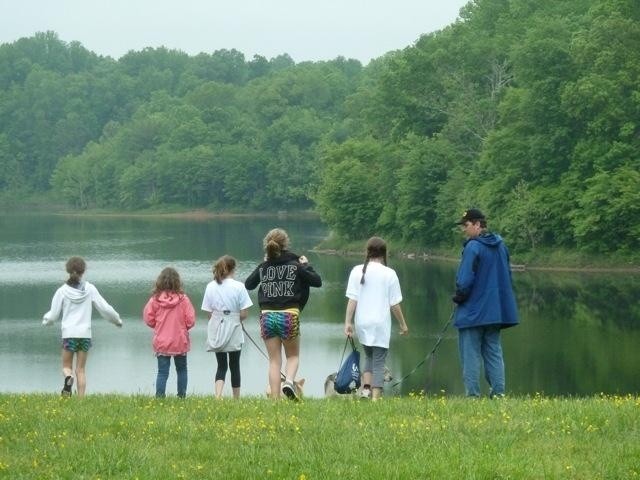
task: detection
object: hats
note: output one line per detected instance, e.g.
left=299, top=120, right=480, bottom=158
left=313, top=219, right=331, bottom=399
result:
left=454, top=209, right=486, bottom=225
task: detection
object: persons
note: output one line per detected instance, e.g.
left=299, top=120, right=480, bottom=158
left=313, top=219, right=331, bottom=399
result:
left=244, top=228, right=322, bottom=404
left=343, top=237, right=407, bottom=402
left=451, top=209, right=520, bottom=400
left=41, top=256, right=122, bottom=400
left=201, top=255, right=253, bottom=401
left=143, top=266, right=196, bottom=399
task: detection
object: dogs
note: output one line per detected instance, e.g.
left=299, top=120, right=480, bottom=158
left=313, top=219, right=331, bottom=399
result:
left=325, top=365, right=393, bottom=399
left=267, top=378, right=305, bottom=401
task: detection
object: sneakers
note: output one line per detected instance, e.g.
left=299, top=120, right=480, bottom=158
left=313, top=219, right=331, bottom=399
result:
left=359, top=389, right=372, bottom=402
left=61, top=375, right=75, bottom=399
left=282, top=380, right=305, bottom=403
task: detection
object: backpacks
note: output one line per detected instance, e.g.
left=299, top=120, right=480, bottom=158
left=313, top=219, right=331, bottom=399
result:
left=334, top=335, right=361, bottom=394
